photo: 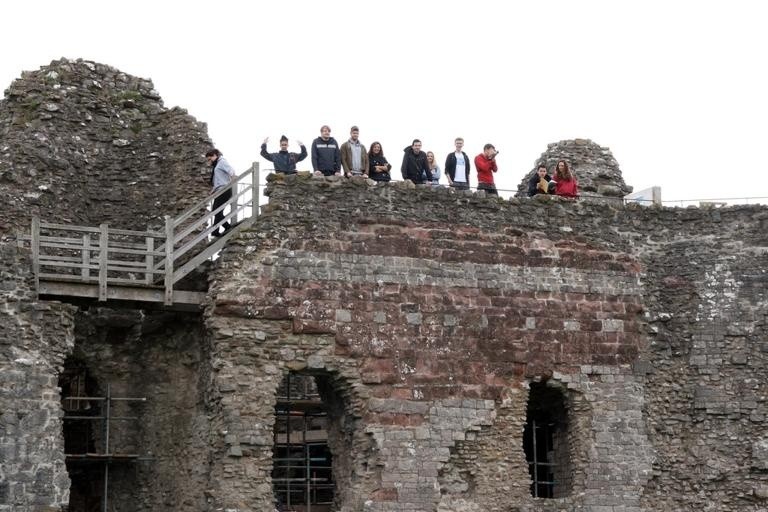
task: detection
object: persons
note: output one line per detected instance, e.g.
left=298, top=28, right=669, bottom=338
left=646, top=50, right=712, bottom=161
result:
left=368, top=142, right=392, bottom=181
left=340, top=126, right=370, bottom=180
left=422, top=152, right=441, bottom=185
left=206, top=149, right=236, bottom=239
left=312, top=126, right=341, bottom=176
left=475, top=144, right=498, bottom=196
left=551, top=160, right=578, bottom=199
left=401, top=140, right=433, bottom=189
left=445, top=137, right=470, bottom=189
left=528, top=162, right=556, bottom=197
left=260, top=135, right=307, bottom=174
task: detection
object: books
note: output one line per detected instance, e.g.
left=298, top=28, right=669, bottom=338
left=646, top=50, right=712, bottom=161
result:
left=539, top=177, right=558, bottom=193
